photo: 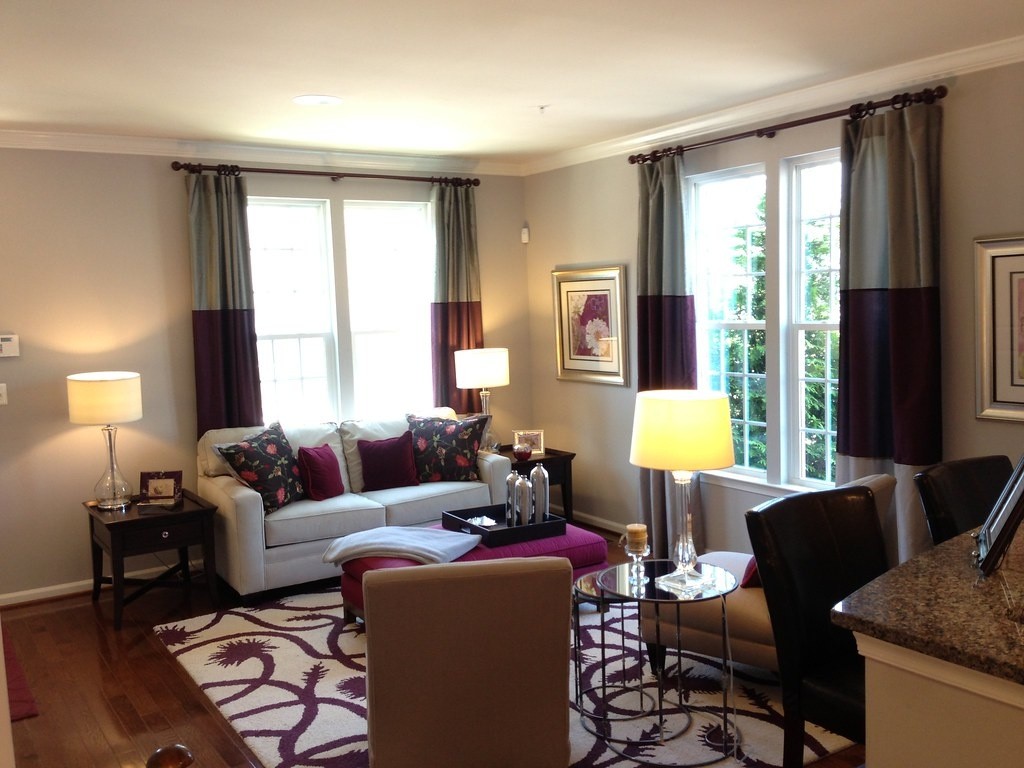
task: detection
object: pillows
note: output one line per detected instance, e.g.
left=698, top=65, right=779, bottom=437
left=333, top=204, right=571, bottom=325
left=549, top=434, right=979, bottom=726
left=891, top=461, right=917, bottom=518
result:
left=211, top=422, right=304, bottom=516
left=299, top=444, right=344, bottom=501
left=741, top=557, right=762, bottom=588
left=357, top=431, right=419, bottom=491
left=406, top=415, right=489, bottom=481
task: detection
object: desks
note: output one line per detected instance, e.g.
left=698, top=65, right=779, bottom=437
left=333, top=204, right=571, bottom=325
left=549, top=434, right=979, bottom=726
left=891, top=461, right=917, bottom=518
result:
left=81, top=489, right=221, bottom=630
left=493, top=444, right=577, bottom=523
left=830, top=524, right=1024, bottom=768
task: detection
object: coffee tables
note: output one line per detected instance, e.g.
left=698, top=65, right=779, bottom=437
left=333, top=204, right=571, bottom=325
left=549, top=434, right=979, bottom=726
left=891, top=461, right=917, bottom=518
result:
left=572, top=559, right=738, bottom=766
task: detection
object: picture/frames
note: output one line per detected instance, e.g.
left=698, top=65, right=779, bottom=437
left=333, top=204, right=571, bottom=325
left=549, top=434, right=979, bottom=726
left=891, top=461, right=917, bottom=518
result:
left=512, top=430, right=545, bottom=454
left=973, top=457, right=1024, bottom=576
left=139, top=470, right=183, bottom=500
left=551, top=266, right=630, bottom=387
left=973, top=235, right=1024, bottom=425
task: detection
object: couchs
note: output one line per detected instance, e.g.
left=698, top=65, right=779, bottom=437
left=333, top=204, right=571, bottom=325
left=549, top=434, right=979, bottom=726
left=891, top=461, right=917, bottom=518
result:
left=361, top=555, right=572, bottom=768
left=196, top=407, right=512, bottom=606
left=639, top=475, right=899, bottom=682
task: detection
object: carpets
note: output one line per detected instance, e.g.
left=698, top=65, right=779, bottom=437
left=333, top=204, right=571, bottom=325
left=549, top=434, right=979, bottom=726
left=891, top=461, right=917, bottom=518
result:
left=1, top=625, right=38, bottom=721
left=153, top=588, right=856, bottom=768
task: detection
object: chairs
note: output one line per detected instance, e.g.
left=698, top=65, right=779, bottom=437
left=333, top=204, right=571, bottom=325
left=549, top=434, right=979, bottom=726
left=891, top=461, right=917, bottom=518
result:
left=744, top=487, right=888, bottom=768
left=913, top=455, right=1013, bottom=546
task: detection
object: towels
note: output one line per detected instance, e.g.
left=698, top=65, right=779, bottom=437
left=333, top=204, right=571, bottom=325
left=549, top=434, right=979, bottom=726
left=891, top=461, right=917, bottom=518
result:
left=322, top=526, right=483, bottom=565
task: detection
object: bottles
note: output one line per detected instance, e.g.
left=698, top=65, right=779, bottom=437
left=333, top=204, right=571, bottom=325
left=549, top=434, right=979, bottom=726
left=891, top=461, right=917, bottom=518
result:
left=515, top=475, right=532, bottom=527
left=530, top=463, right=550, bottom=524
left=506, top=470, right=520, bottom=526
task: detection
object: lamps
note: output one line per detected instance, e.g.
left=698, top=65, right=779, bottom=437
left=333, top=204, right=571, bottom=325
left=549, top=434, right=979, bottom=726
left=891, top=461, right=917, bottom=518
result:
left=629, top=387, right=735, bottom=593
left=454, top=348, right=510, bottom=455
left=65, top=372, right=144, bottom=509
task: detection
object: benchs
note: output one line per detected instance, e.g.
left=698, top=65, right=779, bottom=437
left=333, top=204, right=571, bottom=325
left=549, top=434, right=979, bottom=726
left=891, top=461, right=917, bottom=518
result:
left=341, top=523, right=610, bottom=632
